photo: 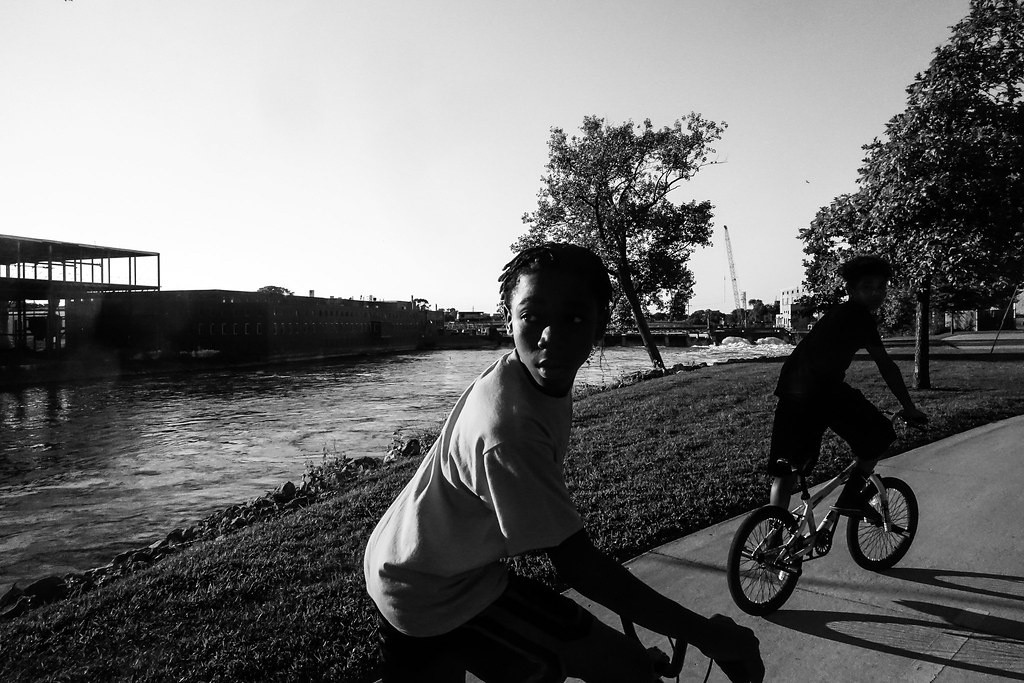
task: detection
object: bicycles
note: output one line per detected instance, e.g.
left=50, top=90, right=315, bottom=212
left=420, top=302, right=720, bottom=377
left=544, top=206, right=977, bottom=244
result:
left=725, top=406, right=929, bottom=618
left=590, top=606, right=723, bottom=682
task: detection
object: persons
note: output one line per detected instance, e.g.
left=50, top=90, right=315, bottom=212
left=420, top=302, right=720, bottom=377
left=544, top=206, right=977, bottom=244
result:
left=755, top=255, right=926, bottom=551
left=359, top=245, right=770, bottom=683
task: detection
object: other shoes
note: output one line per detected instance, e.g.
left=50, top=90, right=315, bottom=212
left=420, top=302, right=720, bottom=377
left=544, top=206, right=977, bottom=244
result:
left=765, top=537, right=784, bottom=549
left=835, top=493, right=883, bottom=520
